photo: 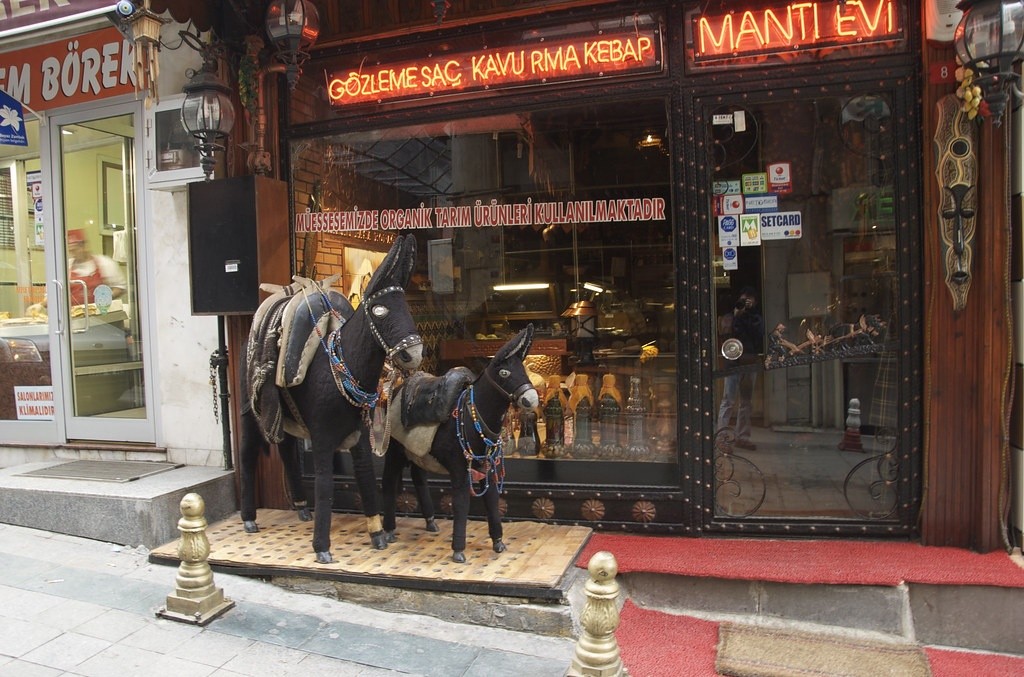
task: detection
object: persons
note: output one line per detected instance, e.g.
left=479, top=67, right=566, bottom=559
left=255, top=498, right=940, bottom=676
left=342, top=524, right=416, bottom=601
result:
left=26, top=228, right=126, bottom=315
left=716, top=287, right=759, bottom=452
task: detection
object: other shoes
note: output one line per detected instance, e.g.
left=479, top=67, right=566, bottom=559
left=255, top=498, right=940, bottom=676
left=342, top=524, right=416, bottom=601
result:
left=734, top=437, right=758, bottom=449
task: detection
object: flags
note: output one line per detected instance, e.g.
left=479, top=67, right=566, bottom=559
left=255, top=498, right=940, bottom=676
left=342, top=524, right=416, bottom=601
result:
left=0, top=89, right=28, bottom=146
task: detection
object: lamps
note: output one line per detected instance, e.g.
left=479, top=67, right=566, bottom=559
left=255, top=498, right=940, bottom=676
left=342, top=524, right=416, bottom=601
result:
left=177, top=30, right=236, bottom=184
left=633, top=127, right=665, bottom=150
left=581, top=279, right=619, bottom=301
left=954, top=0, right=1024, bottom=129
left=264, top=0, right=321, bottom=92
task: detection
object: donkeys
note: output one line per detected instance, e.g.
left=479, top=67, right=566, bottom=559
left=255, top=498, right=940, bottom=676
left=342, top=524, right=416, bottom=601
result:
left=237, top=233, right=421, bottom=563
left=370, top=322, right=540, bottom=563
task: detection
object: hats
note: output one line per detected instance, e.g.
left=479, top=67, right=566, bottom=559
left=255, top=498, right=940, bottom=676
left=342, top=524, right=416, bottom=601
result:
left=65, top=229, right=86, bottom=244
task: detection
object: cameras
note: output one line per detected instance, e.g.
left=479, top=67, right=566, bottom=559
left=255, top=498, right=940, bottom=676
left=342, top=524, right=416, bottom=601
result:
left=736, top=298, right=747, bottom=310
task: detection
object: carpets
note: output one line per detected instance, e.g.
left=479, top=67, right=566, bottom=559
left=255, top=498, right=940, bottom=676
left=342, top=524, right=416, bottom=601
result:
left=711, top=620, right=933, bottom=677
left=577, top=532, right=1024, bottom=588
left=616, top=598, right=1024, bottom=677
